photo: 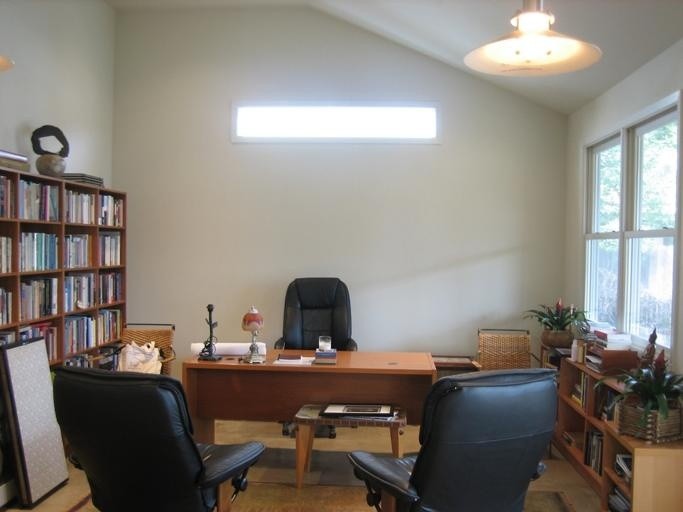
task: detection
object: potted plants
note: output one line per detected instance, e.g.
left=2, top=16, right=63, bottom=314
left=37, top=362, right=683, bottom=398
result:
left=519, top=304, right=589, bottom=349
left=591, top=368, right=682, bottom=444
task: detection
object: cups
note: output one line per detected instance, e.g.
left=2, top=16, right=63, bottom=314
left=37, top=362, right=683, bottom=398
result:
left=319, top=336, right=332, bottom=351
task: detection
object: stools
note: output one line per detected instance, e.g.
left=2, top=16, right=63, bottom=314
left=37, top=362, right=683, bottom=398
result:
left=292, top=403, right=407, bottom=480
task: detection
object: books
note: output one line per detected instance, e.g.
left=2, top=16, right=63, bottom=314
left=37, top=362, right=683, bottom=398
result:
left=585, top=428, right=602, bottom=476
left=0, top=147, right=125, bottom=373
left=593, top=330, right=631, bottom=350
left=278, top=353, right=303, bottom=362
left=607, top=455, right=634, bottom=511
left=562, top=431, right=582, bottom=449
left=571, top=370, right=587, bottom=407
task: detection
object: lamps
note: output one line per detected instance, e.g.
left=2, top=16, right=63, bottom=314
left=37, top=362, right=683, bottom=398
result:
left=462, top=0, right=603, bottom=78
left=238, top=305, right=265, bottom=363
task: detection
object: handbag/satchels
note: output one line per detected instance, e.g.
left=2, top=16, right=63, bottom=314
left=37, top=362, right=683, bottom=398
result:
left=116, top=346, right=163, bottom=374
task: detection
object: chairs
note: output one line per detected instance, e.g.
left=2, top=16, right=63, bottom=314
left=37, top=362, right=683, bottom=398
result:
left=471, top=328, right=558, bottom=371
left=274, top=278, right=356, bottom=439
left=85, top=323, right=176, bottom=376
left=53, top=366, right=266, bottom=512
left=346, top=368, right=557, bottom=512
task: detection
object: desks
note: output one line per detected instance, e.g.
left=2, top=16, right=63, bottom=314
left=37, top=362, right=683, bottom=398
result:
left=182, top=349, right=437, bottom=444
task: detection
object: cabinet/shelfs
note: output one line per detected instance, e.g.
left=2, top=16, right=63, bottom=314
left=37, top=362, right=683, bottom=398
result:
left=539, top=345, right=682, bottom=512
left=0, top=166, right=126, bottom=387
